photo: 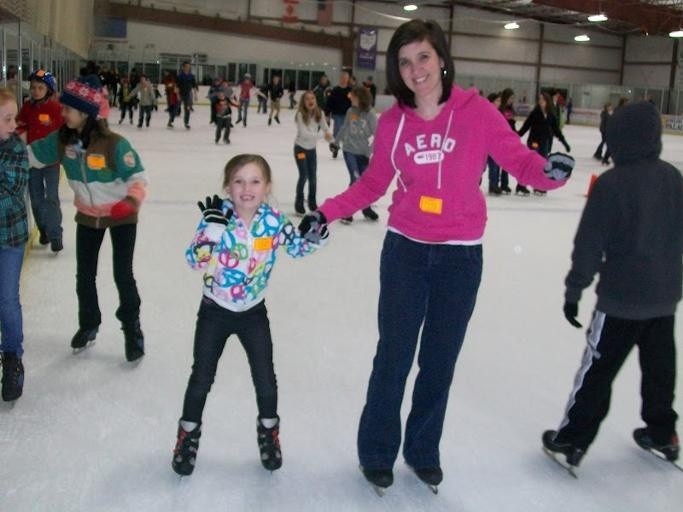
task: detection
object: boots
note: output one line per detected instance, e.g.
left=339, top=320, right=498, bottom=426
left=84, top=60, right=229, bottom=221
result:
left=173, top=419, right=201, bottom=476
left=259, top=418, right=284, bottom=472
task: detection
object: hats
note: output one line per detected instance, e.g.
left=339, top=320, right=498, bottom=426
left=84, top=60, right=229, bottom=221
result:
left=61, top=73, right=102, bottom=119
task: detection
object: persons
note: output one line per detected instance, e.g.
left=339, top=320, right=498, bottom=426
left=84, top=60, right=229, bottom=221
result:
left=0, top=89, right=29, bottom=401
left=471, top=77, right=572, bottom=197
left=27, top=61, right=146, bottom=362
left=309, top=70, right=377, bottom=156
left=172, top=153, right=325, bottom=474
left=21, top=71, right=68, bottom=252
left=299, top=19, right=574, bottom=488
left=293, top=90, right=338, bottom=216
left=542, top=99, right=682, bottom=465
left=594, top=98, right=656, bottom=164
left=85, top=63, right=161, bottom=129
left=162, top=60, right=296, bottom=143
left=336, top=89, right=378, bottom=221
left=0, top=64, right=26, bottom=105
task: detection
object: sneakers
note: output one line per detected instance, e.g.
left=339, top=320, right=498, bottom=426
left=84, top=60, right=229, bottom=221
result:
left=123, top=321, right=145, bottom=362
left=634, top=428, right=679, bottom=461
left=543, top=429, right=584, bottom=468
left=118, top=116, right=280, bottom=145
left=0, top=351, right=25, bottom=402
left=595, top=152, right=610, bottom=167
left=362, top=464, right=394, bottom=485
left=417, top=464, right=443, bottom=487
left=72, top=328, right=99, bottom=348
left=294, top=184, right=548, bottom=221
left=38, top=229, right=63, bottom=251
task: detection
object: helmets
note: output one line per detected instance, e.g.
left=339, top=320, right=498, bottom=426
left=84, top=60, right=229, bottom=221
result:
left=28, top=69, right=58, bottom=93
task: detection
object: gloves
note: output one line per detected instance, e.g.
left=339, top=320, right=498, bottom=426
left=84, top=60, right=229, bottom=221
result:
left=197, top=195, right=233, bottom=227
left=297, top=214, right=328, bottom=249
left=564, top=302, right=583, bottom=328
left=565, top=144, right=570, bottom=151
left=330, top=143, right=339, bottom=157
left=544, top=152, right=576, bottom=181
left=110, top=201, right=134, bottom=221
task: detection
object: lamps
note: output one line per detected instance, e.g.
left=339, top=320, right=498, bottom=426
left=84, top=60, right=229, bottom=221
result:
left=588, top=8, right=609, bottom=22
left=574, top=32, right=591, bottom=41
left=504, top=20, right=520, bottom=30
left=669, top=24, right=683, bottom=38
left=403, top=2, right=418, bottom=12
